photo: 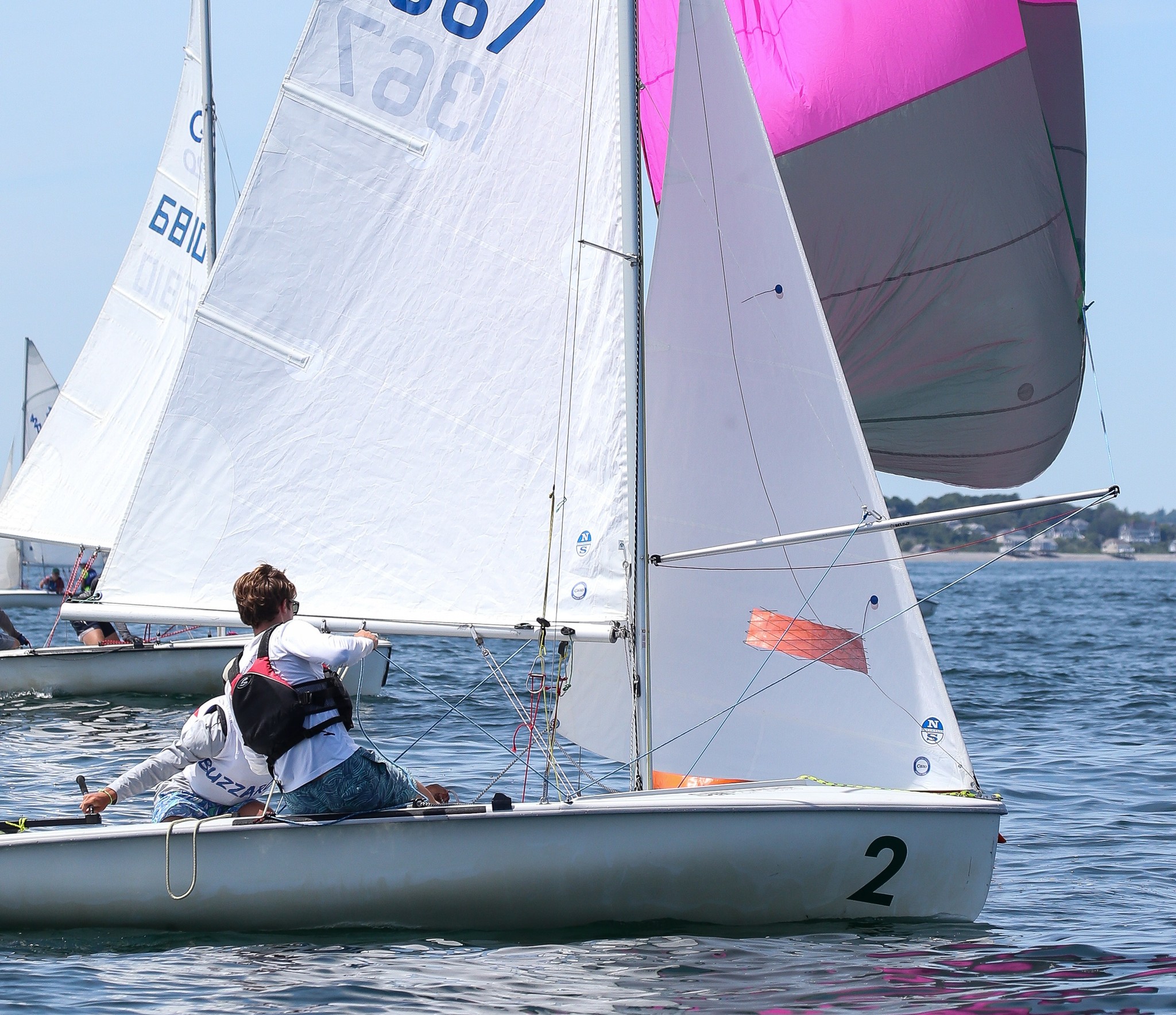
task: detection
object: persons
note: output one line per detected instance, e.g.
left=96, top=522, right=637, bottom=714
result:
left=68, top=574, right=143, bottom=645
left=79, top=559, right=97, bottom=592
left=0, top=608, right=29, bottom=649
left=224, top=565, right=440, bottom=813
left=39, top=567, right=64, bottom=593
left=77, top=694, right=277, bottom=821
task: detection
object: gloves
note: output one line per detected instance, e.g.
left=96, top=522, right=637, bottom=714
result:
left=127, top=635, right=144, bottom=644
left=17, top=634, right=26, bottom=645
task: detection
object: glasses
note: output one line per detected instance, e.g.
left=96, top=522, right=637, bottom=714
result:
left=287, top=598, right=299, bottom=616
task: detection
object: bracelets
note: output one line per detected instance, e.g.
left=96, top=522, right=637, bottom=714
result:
left=98, top=789, right=112, bottom=805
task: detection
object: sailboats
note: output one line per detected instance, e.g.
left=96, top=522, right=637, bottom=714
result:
left=0, top=0, right=392, bottom=695
left=2, top=0, right=1124, bottom=932
